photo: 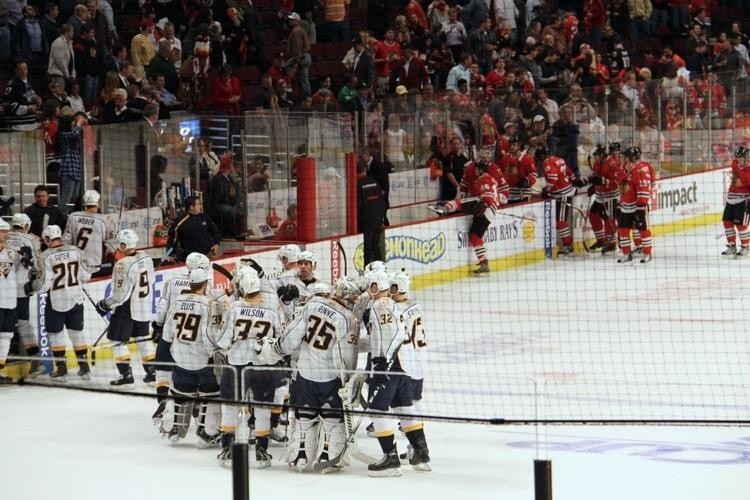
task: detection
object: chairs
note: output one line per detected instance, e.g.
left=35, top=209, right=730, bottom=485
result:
left=203, top=40, right=357, bottom=116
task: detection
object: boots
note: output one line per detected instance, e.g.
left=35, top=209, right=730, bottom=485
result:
left=552, top=238, right=653, bottom=263
left=151, top=397, right=167, bottom=419
left=408, top=441, right=432, bottom=466
left=196, top=419, right=216, bottom=444
left=142, top=366, right=157, bottom=383
left=109, top=366, right=135, bottom=385
left=367, top=442, right=401, bottom=472
left=167, top=421, right=179, bottom=439
left=217, top=426, right=341, bottom=462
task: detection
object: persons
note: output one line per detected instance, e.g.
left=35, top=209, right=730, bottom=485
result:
left=8, top=213, right=46, bottom=378
left=0, top=218, right=18, bottom=383
left=41, top=224, right=92, bottom=380
left=1, top=0, right=750, bottom=281
left=152, top=243, right=431, bottom=478
left=96, top=229, right=157, bottom=386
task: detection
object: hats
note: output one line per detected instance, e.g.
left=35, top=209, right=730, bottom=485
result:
left=190, top=270, right=211, bottom=284
left=218, top=157, right=233, bottom=174
left=533, top=115, right=546, bottom=123
left=504, top=122, right=516, bottom=129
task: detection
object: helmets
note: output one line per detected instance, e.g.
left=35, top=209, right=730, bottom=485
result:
left=82, top=189, right=100, bottom=206
left=305, top=260, right=412, bottom=305
left=297, top=250, right=317, bottom=269
left=276, top=243, right=302, bottom=267
left=475, top=132, right=550, bottom=171
left=41, top=225, right=62, bottom=248
left=234, top=265, right=262, bottom=297
left=12, top=212, right=32, bottom=229
left=185, top=251, right=210, bottom=270
left=590, top=141, right=641, bottom=162
left=116, top=229, right=139, bottom=253
left=0, top=217, right=11, bottom=232
left=729, top=147, right=749, bottom=166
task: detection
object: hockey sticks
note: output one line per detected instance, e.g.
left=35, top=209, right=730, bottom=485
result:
left=315, top=346, right=400, bottom=472
left=91, top=248, right=172, bottom=366
left=588, top=155, right=614, bottom=255
left=582, top=196, right=592, bottom=253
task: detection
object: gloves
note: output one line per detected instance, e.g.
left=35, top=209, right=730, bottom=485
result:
left=95, top=299, right=111, bottom=317
left=586, top=187, right=595, bottom=197
left=339, top=382, right=362, bottom=407
left=517, top=178, right=528, bottom=189
left=16, top=245, right=35, bottom=269
left=470, top=200, right=487, bottom=218
left=281, top=285, right=300, bottom=303
left=254, top=336, right=285, bottom=365
left=23, top=279, right=42, bottom=296
left=591, top=176, right=603, bottom=184
left=573, top=176, right=590, bottom=188
left=636, top=209, right=646, bottom=219
left=150, top=321, right=163, bottom=343
left=541, top=187, right=554, bottom=201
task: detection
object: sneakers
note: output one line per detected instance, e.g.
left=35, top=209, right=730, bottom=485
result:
left=474, top=263, right=489, bottom=275
left=49, top=364, right=67, bottom=379
left=735, top=247, right=749, bottom=256
left=77, top=363, right=91, bottom=377
left=428, top=203, right=445, bottom=215
left=721, top=248, right=737, bottom=256
